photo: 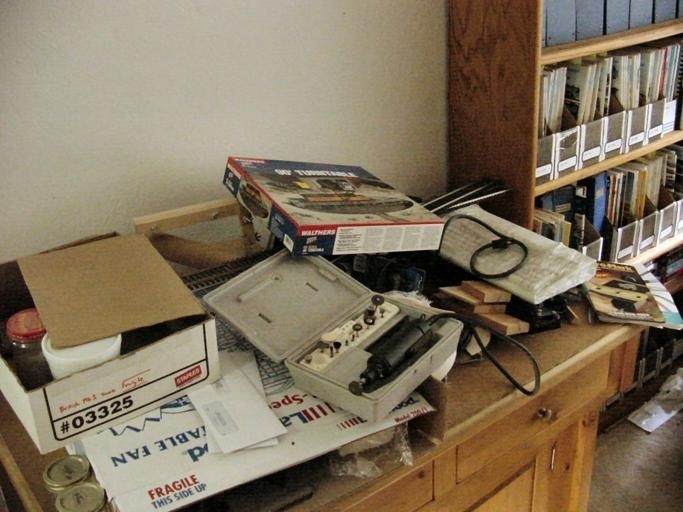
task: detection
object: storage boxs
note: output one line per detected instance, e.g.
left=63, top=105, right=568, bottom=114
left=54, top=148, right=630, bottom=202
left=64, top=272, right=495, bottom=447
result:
left=1, top=230, right=217, bottom=457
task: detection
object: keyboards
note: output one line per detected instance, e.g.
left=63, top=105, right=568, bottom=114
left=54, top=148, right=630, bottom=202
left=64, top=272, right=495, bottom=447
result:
left=436, top=202, right=599, bottom=307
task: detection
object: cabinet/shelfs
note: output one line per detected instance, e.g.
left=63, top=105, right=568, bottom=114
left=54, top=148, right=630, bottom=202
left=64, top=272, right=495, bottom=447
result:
left=443, top=3, right=683, bottom=437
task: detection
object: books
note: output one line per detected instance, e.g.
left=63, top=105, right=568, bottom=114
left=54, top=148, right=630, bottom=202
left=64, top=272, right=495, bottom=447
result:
left=585, top=260, right=683, bottom=331
left=533, top=145, right=683, bottom=248
left=539, top=43, right=683, bottom=138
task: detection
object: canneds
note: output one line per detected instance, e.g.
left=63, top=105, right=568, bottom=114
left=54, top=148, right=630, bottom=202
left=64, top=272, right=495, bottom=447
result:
left=55, top=483, right=107, bottom=511
left=42, top=455, right=90, bottom=493
left=5, top=307, right=54, bottom=391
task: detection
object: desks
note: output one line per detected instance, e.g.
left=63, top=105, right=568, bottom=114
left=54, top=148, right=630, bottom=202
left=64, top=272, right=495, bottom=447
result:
left=0, top=298, right=649, bottom=512
